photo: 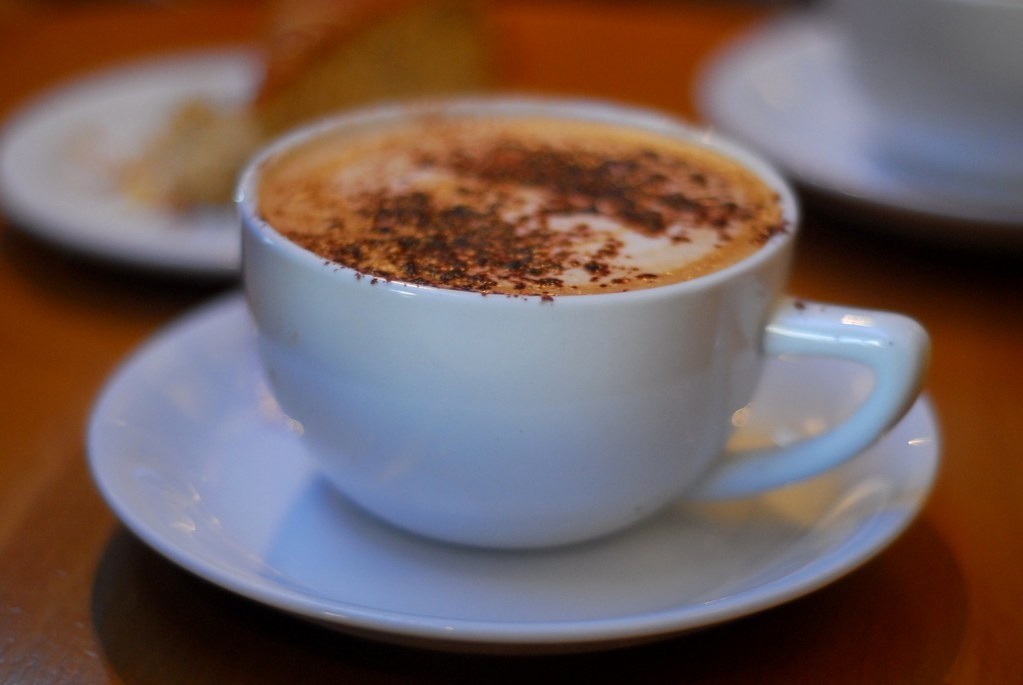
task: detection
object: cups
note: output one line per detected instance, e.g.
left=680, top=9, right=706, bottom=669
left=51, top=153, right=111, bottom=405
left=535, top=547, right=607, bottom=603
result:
left=236, top=97, right=928, bottom=549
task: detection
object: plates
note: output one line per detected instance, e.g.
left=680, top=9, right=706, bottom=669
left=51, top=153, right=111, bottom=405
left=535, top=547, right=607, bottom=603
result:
left=89, top=298, right=941, bottom=645
left=695, top=21, right=1023, bottom=223
left=0, top=52, right=260, bottom=271
